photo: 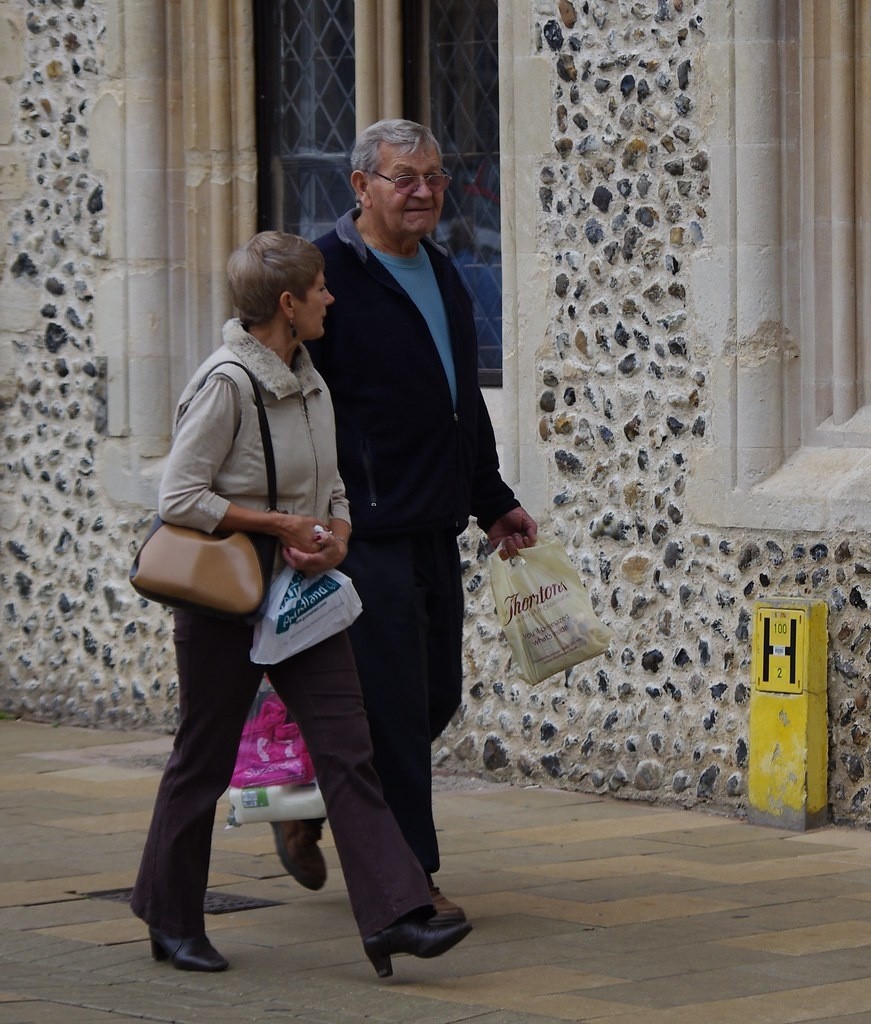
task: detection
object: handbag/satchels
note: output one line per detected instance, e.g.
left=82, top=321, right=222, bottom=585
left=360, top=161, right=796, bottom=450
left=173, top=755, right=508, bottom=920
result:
left=129, top=508, right=279, bottom=620
left=251, top=525, right=364, bottom=667
left=487, top=531, right=612, bottom=688
left=225, top=674, right=327, bottom=830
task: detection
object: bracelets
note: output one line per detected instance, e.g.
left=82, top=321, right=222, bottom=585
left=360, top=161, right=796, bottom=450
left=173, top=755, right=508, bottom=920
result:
left=334, top=534, right=348, bottom=545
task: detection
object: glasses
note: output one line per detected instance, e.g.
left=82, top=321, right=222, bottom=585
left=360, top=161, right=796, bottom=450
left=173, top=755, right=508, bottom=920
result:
left=372, top=170, right=451, bottom=196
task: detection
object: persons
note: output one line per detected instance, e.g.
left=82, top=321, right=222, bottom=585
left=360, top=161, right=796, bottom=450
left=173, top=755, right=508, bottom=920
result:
left=270, top=122, right=536, bottom=922
left=128, top=229, right=472, bottom=982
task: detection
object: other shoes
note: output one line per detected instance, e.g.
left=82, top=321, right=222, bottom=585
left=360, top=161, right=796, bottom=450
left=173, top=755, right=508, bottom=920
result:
left=413, top=871, right=469, bottom=928
left=269, top=820, right=327, bottom=891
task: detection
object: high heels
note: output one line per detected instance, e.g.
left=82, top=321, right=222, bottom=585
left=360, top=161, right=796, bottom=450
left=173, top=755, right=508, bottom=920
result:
left=361, top=914, right=474, bottom=981
left=147, top=919, right=230, bottom=972
